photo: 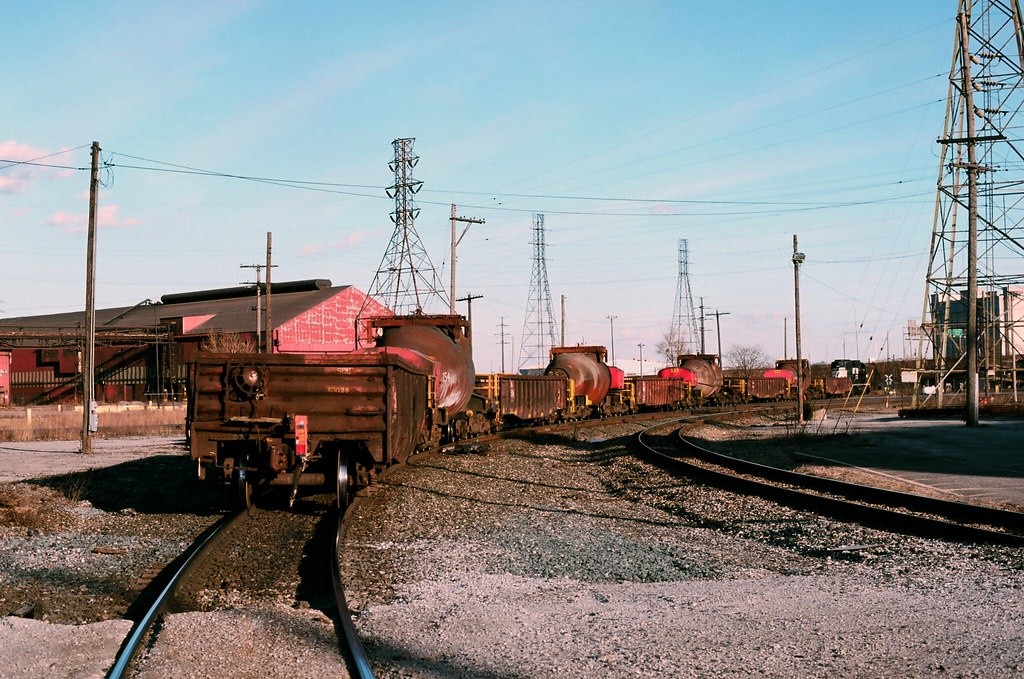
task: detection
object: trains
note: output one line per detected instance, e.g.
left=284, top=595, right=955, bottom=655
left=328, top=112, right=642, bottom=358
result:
left=184, top=308, right=873, bottom=520
